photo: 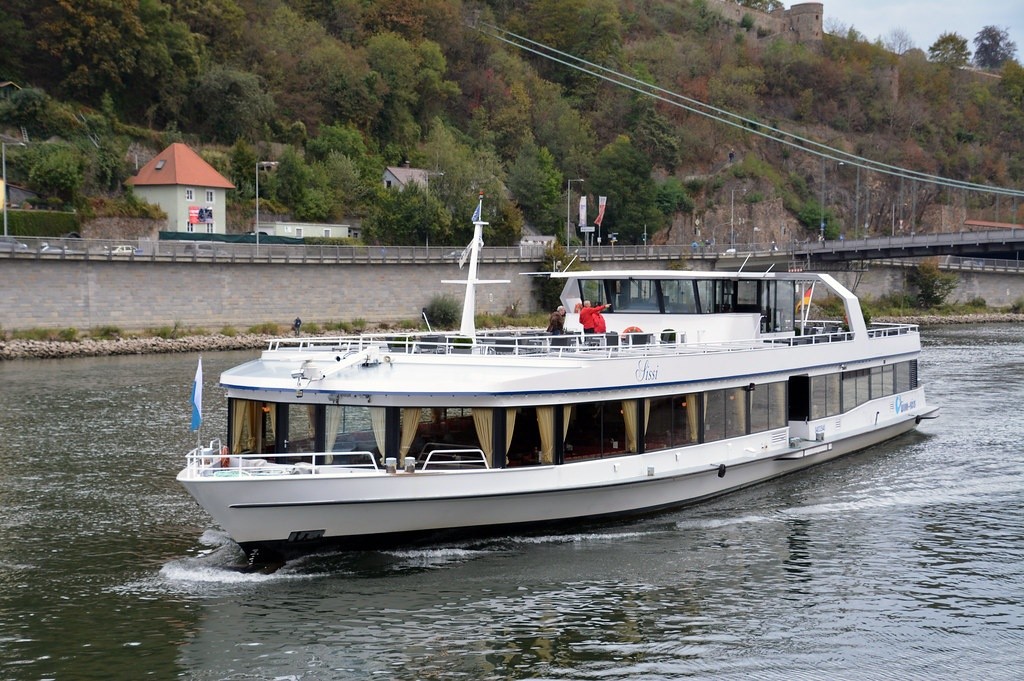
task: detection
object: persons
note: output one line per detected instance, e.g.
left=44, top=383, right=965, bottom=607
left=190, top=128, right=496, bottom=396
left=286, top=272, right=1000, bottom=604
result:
left=546, top=306, right=566, bottom=335
left=294, top=317, right=301, bottom=336
left=579, top=300, right=611, bottom=351
left=818, top=235, right=825, bottom=249
left=381, top=247, right=385, bottom=262
left=770, top=234, right=776, bottom=250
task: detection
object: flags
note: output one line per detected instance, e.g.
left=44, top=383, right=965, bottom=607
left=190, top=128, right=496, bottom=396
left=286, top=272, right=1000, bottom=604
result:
left=795, top=283, right=814, bottom=315
left=471, top=203, right=480, bottom=222
left=188, top=359, right=202, bottom=432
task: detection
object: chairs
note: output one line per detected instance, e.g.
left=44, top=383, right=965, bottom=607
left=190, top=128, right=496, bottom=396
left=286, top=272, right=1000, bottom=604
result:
left=419, top=331, right=655, bottom=358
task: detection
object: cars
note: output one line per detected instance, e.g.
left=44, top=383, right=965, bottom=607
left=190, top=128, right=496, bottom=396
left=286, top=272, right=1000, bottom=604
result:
left=38, top=244, right=67, bottom=253
left=101, top=245, right=138, bottom=257
left=1, top=236, right=28, bottom=249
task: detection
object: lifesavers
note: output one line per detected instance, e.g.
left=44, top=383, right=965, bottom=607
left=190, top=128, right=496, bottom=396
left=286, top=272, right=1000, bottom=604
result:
left=575, top=304, right=583, bottom=313
left=623, top=326, right=642, bottom=333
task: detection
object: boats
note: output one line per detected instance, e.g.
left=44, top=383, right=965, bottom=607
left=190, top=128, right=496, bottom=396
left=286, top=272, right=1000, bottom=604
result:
left=176, top=187, right=941, bottom=569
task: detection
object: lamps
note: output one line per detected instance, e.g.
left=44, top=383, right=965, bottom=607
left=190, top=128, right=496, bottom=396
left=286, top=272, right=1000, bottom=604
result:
left=290, top=369, right=304, bottom=397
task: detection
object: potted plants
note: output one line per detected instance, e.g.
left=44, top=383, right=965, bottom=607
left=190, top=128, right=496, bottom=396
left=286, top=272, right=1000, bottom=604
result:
left=390, top=336, right=414, bottom=354
left=450, top=336, right=473, bottom=355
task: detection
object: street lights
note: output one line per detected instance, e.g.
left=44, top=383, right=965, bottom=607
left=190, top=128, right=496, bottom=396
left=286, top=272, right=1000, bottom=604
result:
left=255, top=162, right=281, bottom=258
left=568, top=178, right=585, bottom=254
left=891, top=203, right=909, bottom=237
left=732, top=188, right=746, bottom=249
